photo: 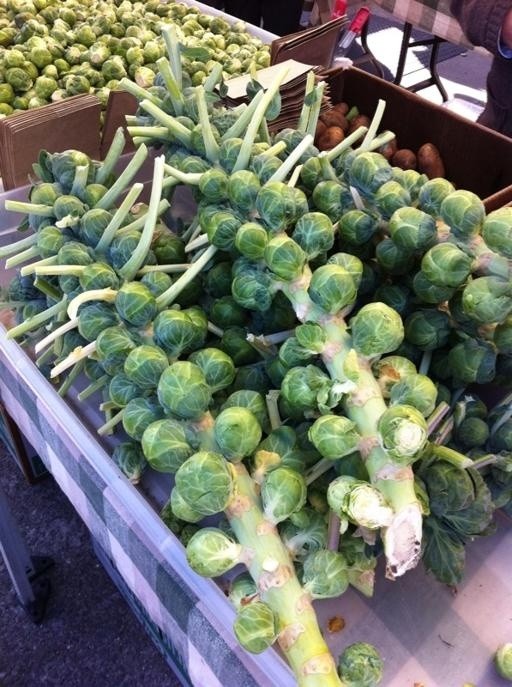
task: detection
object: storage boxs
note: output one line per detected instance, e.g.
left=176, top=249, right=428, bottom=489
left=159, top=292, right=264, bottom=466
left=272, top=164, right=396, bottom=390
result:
left=313, top=61, right=512, bottom=214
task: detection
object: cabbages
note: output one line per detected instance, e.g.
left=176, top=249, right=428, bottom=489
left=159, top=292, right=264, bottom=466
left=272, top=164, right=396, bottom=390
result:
left=1, top=0, right=275, bottom=112
left=0, top=30, right=512, bottom=687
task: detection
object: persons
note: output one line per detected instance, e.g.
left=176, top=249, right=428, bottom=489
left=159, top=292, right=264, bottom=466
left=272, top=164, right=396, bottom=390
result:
left=223, top=0, right=305, bottom=38
left=451, top=1, right=512, bottom=138
